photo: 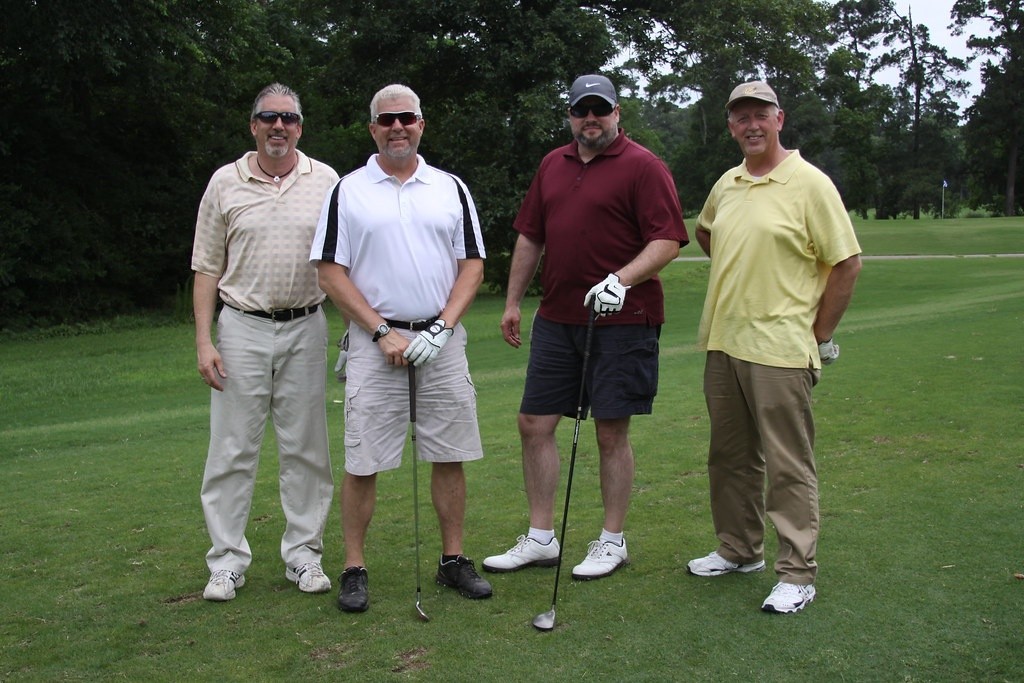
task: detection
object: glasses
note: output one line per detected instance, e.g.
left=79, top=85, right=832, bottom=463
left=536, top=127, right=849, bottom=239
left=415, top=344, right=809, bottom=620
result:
left=253, top=110, right=300, bottom=124
left=373, top=112, right=422, bottom=126
left=569, top=103, right=616, bottom=118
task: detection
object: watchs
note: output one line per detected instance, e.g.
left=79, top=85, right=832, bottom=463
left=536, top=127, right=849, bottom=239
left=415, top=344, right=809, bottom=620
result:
left=372, top=322, right=392, bottom=342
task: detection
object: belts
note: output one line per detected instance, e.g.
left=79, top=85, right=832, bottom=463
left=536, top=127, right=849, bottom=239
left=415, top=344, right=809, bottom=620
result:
left=224, top=301, right=318, bottom=323
left=382, top=314, right=441, bottom=331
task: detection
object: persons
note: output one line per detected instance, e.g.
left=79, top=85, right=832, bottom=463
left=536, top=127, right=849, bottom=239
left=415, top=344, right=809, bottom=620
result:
left=308, top=85, right=493, bottom=614
left=190, top=84, right=352, bottom=601
left=687, top=80, right=862, bottom=614
left=482, top=75, right=690, bottom=579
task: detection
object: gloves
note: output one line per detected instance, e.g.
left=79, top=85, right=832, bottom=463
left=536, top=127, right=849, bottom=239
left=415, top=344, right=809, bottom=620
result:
left=334, top=330, right=350, bottom=373
left=818, top=337, right=840, bottom=365
left=584, top=272, right=631, bottom=320
left=403, top=318, right=454, bottom=367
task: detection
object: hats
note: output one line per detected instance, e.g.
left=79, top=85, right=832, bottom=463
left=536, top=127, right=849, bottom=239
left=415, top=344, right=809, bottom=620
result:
left=725, top=81, right=779, bottom=117
left=569, top=74, right=617, bottom=108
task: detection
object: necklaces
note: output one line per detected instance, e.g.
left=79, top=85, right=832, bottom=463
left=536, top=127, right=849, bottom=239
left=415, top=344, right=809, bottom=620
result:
left=256, top=157, right=295, bottom=182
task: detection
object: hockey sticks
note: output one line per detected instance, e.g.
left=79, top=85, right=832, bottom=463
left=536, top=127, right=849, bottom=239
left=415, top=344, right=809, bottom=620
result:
left=408, top=360, right=430, bottom=622
left=533, top=294, right=599, bottom=631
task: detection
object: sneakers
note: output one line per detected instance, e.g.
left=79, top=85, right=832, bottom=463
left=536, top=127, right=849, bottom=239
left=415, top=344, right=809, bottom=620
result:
left=435, top=553, right=492, bottom=599
left=482, top=534, right=560, bottom=573
left=687, top=551, right=766, bottom=577
left=286, top=562, right=331, bottom=593
left=337, top=565, right=369, bottom=612
left=572, top=538, right=628, bottom=579
left=761, top=581, right=815, bottom=613
left=203, top=570, right=245, bottom=601
